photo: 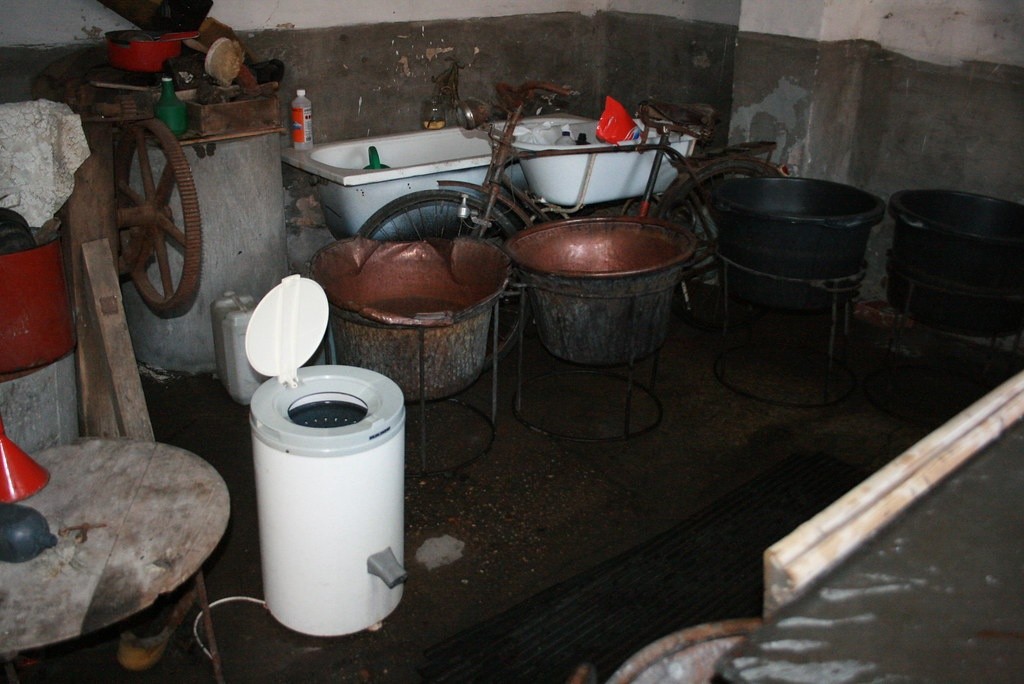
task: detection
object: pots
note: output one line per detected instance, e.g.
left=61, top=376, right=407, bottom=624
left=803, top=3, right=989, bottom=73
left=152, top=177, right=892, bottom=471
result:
left=885, top=188, right=1024, bottom=337
left=701, top=176, right=885, bottom=278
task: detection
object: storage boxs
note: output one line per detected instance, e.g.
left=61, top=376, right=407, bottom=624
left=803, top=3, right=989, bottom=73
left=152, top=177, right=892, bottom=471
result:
left=185, top=95, right=282, bottom=137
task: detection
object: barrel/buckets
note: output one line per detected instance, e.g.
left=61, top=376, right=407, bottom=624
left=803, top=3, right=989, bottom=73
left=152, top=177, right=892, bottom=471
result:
left=211, top=289, right=271, bottom=406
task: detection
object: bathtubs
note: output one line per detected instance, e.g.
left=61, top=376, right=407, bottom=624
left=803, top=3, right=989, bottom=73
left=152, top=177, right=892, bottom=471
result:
left=490, top=112, right=705, bottom=206
left=280, top=110, right=599, bottom=240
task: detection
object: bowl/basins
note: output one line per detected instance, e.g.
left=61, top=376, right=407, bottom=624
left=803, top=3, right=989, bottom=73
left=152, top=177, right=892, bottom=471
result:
left=104, top=30, right=181, bottom=73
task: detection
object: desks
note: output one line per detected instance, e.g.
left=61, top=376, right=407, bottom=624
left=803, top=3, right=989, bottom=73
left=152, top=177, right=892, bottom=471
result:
left=0, top=435, right=233, bottom=684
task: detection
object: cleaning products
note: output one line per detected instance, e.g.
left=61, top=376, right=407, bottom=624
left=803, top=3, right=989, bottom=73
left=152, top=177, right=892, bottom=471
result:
left=292, top=90, right=314, bottom=152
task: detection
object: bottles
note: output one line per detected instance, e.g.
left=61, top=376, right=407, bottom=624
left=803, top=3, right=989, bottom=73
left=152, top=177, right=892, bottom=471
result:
left=291, top=88, right=313, bottom=150
left=555, top=131, right=590, bottom=146
left=156, top=78, right=187, bottom=136
